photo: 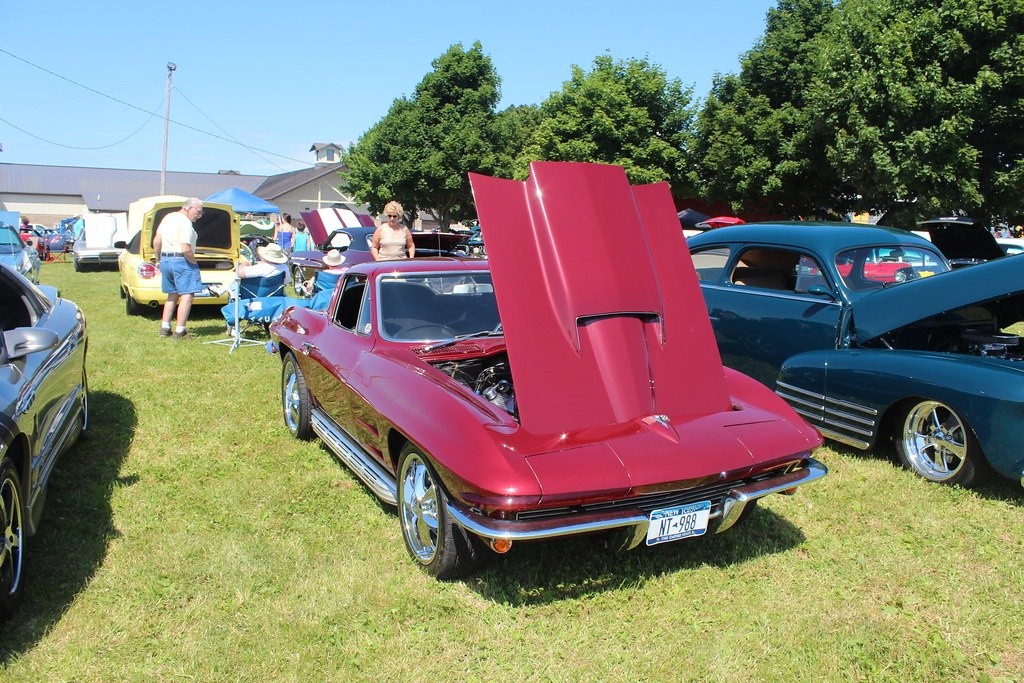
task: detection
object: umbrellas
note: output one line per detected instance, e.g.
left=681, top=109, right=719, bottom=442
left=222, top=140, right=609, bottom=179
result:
left=679, top=208, right=746, bottom=227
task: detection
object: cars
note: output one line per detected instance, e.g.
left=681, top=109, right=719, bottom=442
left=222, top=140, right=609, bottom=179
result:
left=287, top=208, right=471, bottom=296
left=0, top=264, right=90, bottom=620
left=20, top=224, right=58, bottom=237
left=72, top=228, right=121, bottom=272
left=0, top=211, right=41, bottom=284
left=894, top=221, right=1024, bottom=283
left=694, top=217, right=911, bottom=283
left=267, top=161, right=828, bottom=583
left=114, top=195, right=251, bottom=315
left=686, top=221, right=1024, bottom=489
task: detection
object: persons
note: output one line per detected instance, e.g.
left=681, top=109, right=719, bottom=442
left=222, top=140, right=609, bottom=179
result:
left=73, top=215, right=85, bottom=241
left=371, top=201, right=415, bottom=261
left=20, top=216, right=29, bottom=234
left=227, top=243, right=290, bottom=336
left=307, top=249, right=349, bottom=296
left=154, top=198, right=203, bottom=339
left=273, top=212, right=311, bottom=253
left=204, top=186, right=282, bottom=227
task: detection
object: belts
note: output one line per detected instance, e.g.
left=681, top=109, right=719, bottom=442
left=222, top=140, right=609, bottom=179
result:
left=161, top=252, right=184, bottom=257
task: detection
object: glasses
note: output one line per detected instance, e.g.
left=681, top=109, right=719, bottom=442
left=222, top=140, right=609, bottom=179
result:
left=388, top=214, right=398, bottom=219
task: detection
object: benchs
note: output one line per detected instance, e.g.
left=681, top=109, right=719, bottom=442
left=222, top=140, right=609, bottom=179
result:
left=335, top=282, right=494, bottom=329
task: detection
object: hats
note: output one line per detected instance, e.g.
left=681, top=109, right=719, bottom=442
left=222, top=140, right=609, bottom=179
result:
left=256, top=242, right=289, bottom=264
left=322, top=249, right=347, bottom=267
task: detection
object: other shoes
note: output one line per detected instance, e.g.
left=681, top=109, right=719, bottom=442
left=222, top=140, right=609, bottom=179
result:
left=159, top=325, right=173, bottom=338
left=172, top=329, right=198, bottom=340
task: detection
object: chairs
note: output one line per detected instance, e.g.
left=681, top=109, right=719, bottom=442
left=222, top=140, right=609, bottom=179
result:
left=44, top=235, right=66, bottom=263
left=68, top=233, right=76, bottom=251
left=226, top=271, right=286, bottom=348
left=311, top=271, right=343, bottom=300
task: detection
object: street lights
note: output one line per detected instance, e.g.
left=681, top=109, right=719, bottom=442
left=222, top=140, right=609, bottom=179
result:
left=160, top=62, right=177, bottom=196
